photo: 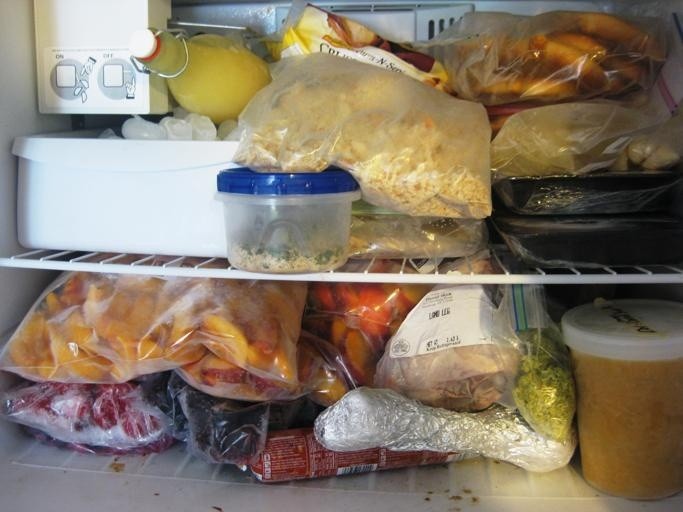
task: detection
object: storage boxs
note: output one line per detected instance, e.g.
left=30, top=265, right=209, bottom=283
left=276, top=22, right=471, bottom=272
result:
left=12, top=125, right=248, bottom=261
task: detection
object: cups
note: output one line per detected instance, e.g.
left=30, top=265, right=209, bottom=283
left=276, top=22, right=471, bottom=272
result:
left=560, top=299, right=682, bottom=500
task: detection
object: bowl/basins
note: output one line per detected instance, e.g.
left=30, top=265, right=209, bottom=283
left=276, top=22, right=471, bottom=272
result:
left=216, top=165, right=363, bottom=275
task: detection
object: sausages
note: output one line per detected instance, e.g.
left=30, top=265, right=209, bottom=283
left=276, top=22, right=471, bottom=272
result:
left=249, top=427, right=480, bottom=483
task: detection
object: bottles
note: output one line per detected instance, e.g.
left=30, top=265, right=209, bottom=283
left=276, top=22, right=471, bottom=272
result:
left=126, top=25, right=272, bottom=124
